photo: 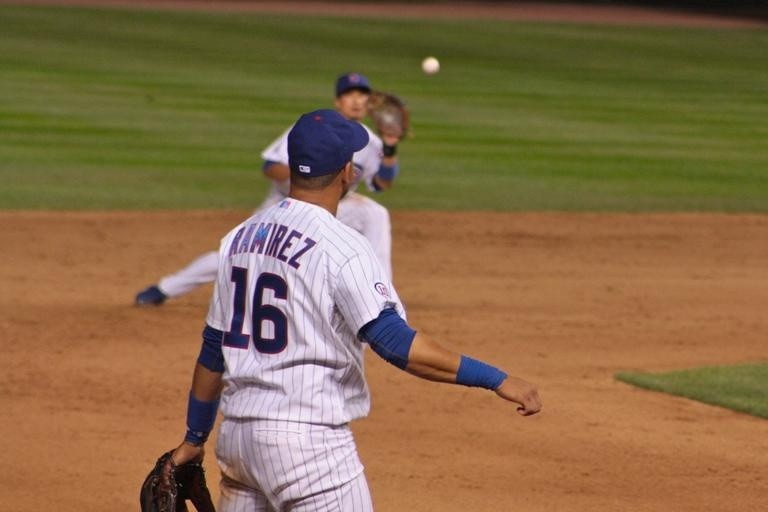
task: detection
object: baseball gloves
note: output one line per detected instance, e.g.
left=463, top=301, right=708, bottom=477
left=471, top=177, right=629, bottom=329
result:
left=365, top=89, right=410, bottom=141
left=141, top=449, right=216, bottom=511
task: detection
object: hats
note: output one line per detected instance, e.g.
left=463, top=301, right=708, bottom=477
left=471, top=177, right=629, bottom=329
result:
left=336, top=73, right=370, bottom=97
left=288, top=109, right=369, bottom=178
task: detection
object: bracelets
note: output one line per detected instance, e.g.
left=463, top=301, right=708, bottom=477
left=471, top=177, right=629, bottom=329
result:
left=182, top=390, right=221, bottom=447
left=452, top=354, right=509, bottom=392
left=382, top=141, right=398, bottom=158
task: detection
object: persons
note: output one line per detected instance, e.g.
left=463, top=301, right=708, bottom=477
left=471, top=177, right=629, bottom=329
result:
left=135, top=68, right=409, bottom=331
left=159, top=107, right=544, bottom=512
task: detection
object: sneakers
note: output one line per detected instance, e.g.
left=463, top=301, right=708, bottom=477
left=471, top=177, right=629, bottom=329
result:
left=137, top=287, right=165, bottom=306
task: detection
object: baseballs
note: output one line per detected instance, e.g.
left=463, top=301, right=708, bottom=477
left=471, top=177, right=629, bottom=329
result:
left=420, top=56, right=440, bottom=75
